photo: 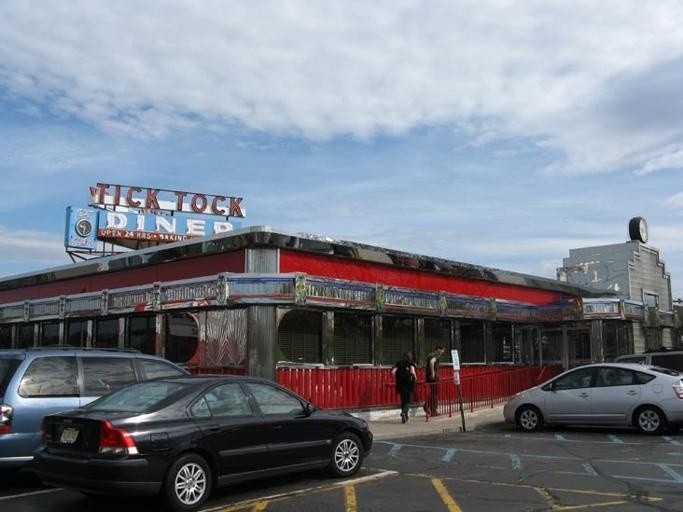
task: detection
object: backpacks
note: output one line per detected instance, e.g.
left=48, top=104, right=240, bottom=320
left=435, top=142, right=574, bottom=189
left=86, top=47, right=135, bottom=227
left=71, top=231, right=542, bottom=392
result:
left=395, top=360, right=414, bottom=385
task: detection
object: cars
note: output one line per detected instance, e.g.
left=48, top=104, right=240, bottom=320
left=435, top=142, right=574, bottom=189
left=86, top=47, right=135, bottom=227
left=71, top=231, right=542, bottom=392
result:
left=503, top=350, right=683, bottom=434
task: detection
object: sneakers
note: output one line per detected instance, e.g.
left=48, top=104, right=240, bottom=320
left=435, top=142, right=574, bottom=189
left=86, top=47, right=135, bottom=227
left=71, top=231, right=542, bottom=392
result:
left=399, top=405, right=441, bottom=424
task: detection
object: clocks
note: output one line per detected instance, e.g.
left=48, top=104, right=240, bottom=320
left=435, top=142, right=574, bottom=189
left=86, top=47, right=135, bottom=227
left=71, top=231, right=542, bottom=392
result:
left=629, top=217, right=649, bottom=243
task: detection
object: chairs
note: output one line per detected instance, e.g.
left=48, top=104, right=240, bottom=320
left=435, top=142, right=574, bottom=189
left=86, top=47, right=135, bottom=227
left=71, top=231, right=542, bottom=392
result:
left=167, top=387, right=242, bottom=418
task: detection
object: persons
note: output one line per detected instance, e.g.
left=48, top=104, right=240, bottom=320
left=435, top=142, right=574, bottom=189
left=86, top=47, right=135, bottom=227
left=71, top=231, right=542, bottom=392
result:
left=389, top=352, right=417, bottom=424
left=422, top=342, right=445, bottom=416
